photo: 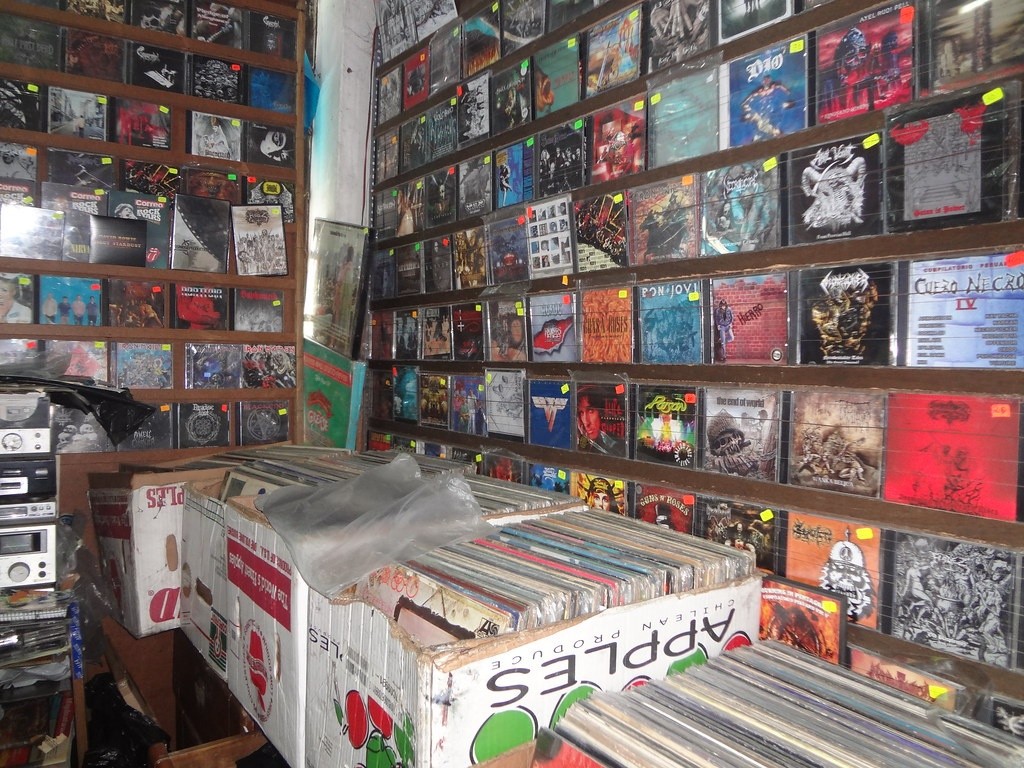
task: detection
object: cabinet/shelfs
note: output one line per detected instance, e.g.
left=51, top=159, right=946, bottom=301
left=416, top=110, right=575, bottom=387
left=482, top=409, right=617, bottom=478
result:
left=0, top=0, right=306, bottom=768
left=357, top=0, right=1024, bottom=704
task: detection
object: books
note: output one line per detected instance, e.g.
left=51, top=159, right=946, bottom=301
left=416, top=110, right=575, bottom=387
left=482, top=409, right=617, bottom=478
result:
left=0, top=0, right=1024, bottom=768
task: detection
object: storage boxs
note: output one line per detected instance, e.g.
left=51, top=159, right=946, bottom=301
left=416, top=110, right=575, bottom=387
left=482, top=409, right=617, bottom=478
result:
left=179, top=477, right=587, bottom=768
left=302, top=336, right=366, bottom=455
left=306, top=568, right=763, bottom=768
left=89, top=461, right=237, bottom=640
